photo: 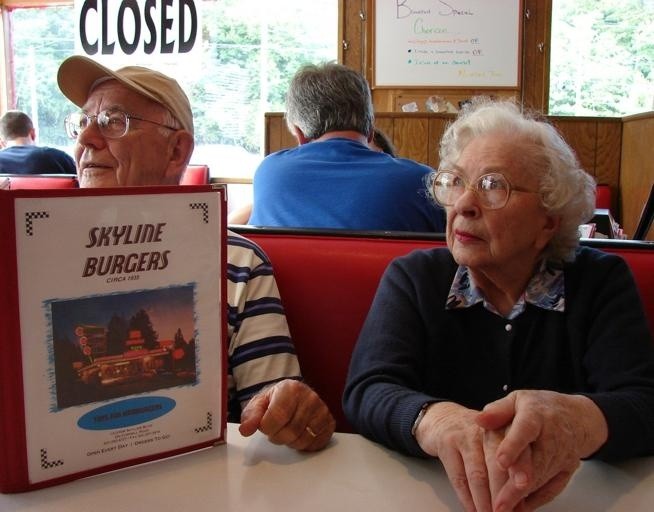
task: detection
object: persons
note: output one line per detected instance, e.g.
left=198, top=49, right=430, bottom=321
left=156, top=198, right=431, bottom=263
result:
left=368, top=128, right=396, bottom=161
left=57, top=53, right=337, bottom=453
left=340, top=100, right=654, bottom=510
left=248, top=58, right=447, bottom=231
left=0, top=110, right=78, bottom=178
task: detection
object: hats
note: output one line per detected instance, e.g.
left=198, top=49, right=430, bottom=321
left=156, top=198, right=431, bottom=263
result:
left=57, top=56, right=193, bottom=138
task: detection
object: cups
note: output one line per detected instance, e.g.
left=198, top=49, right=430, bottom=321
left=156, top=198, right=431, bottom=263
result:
left=578, top=225, right=592, bottom=238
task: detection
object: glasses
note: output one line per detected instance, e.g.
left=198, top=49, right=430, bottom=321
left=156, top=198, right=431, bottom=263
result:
left=65, top=111, right=180, bottom=138
left=432, top=171, right=545, bottom=209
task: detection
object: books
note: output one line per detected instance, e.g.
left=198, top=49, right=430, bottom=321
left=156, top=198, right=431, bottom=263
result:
left=1, top=177, right=228, bottom=492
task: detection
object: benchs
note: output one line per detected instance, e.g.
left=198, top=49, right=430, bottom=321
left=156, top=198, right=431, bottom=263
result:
left=596, top=183, right=611, bottom=209
left=228, top=225, right=654, bottom=436
left=0, top=173, right=77, bottom=190
left=179, top=166, right=210, bottom=185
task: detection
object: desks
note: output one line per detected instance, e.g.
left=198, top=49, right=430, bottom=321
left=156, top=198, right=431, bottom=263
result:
left=0, top=421, right=654, bottom=512
